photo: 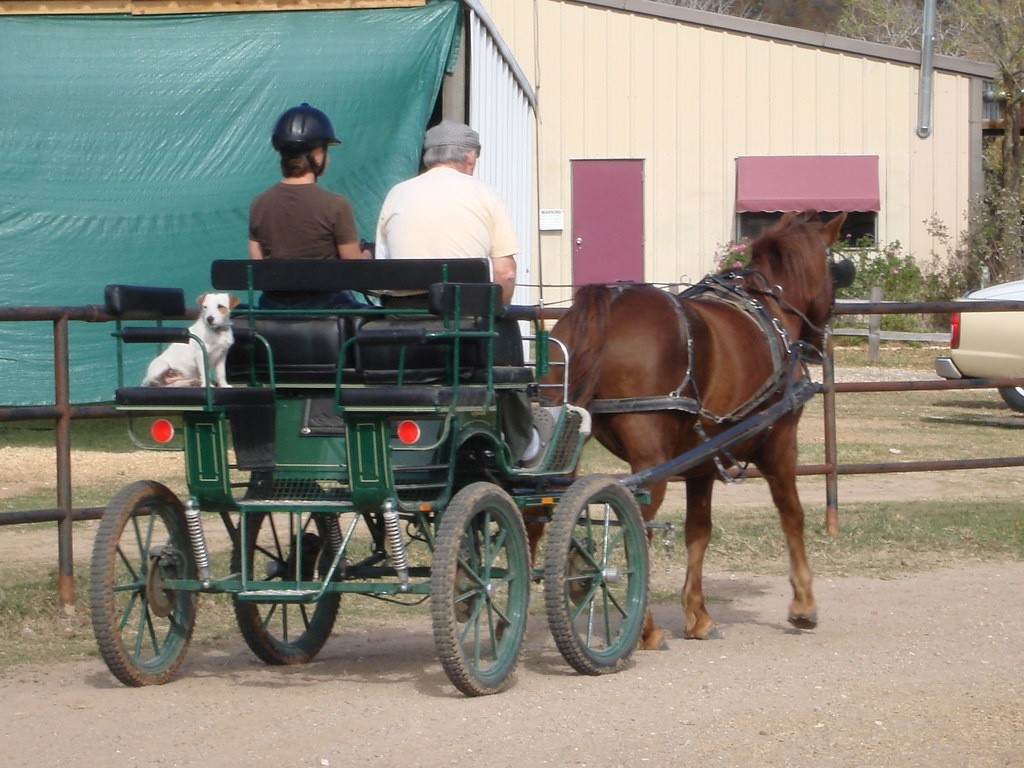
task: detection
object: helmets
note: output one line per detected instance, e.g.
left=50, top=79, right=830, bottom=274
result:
left=272, top=103, right=342, bottom=146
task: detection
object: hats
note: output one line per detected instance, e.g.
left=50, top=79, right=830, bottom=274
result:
left=424, top=120, right=481, bottom=157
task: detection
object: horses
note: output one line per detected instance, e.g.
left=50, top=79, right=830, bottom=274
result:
left=494, top=209, right=848, bottom=651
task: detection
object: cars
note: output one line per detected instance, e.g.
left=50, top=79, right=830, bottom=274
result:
left=931, top=279, right=1024, bottom=418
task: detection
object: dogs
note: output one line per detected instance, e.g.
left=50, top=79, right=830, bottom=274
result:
left=140, top=293, right=241, bottom=388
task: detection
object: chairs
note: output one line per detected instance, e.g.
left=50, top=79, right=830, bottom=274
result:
left=104, top=281, right=276, bottom=449
left=335, top=281, right=502, bottom=452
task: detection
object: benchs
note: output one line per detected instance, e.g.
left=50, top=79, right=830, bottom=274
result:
left=212, top=258, right=536, bottom=382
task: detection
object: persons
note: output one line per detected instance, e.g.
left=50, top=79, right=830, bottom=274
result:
left=375, top=120, right=554, bottom=470
left=247, top=103, right=375, bottom=320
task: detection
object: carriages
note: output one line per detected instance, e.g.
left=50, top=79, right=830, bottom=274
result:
left=79, top=205, right=850, bottom=699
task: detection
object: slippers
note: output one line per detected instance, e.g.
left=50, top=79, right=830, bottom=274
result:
left=518, top=406, right=555, bottom=468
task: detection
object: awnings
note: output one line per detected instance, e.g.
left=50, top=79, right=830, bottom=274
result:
left=734, top=154, right=881, bottom=214
